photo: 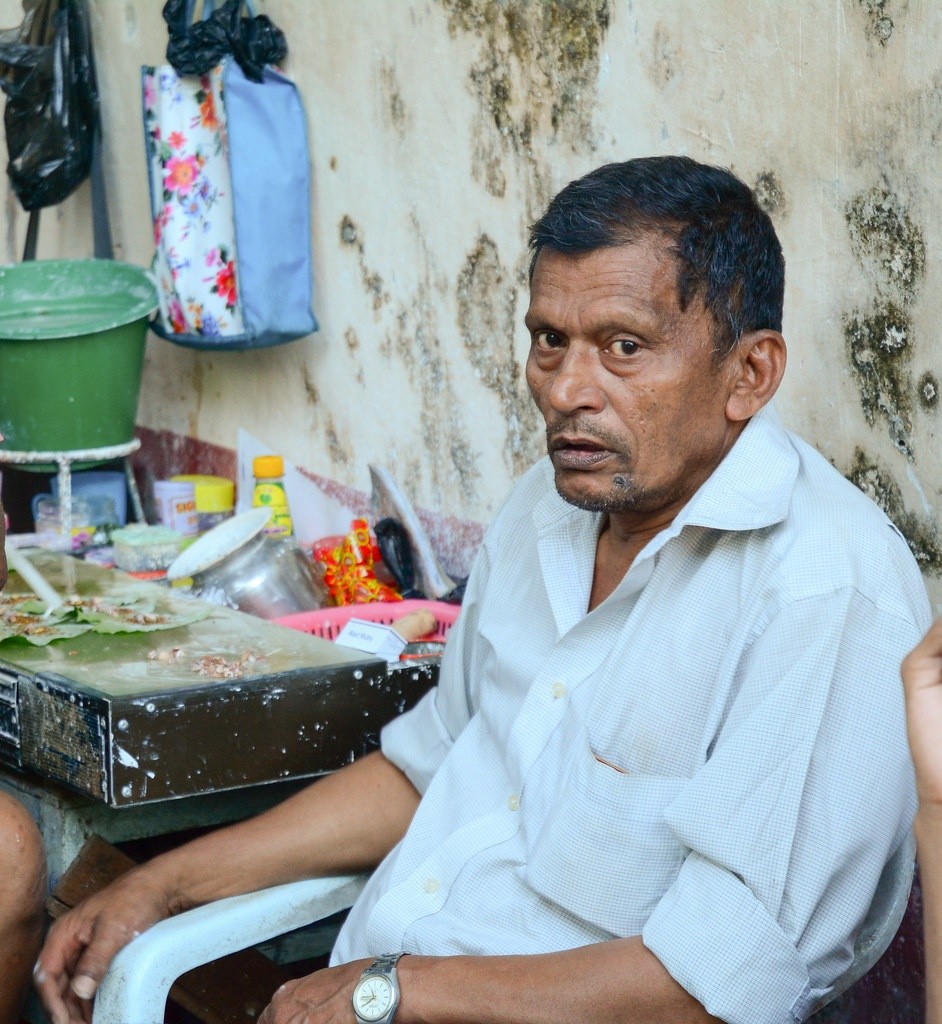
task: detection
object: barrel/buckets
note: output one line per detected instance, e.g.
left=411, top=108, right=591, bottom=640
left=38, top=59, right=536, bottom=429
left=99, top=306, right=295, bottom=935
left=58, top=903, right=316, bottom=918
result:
left=0, top=258, right=160, bottom=475
left=50, top=472, right=126, bottom=526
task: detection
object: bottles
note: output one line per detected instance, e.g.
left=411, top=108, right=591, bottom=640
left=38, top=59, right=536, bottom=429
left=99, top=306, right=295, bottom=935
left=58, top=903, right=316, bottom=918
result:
left=165, top=508, right=329, bottom=622
left=154, top=481, right=200, bottom=593
left=36, top=497, right=120, bottom=558
left=251, top=453, right=294, bottom=543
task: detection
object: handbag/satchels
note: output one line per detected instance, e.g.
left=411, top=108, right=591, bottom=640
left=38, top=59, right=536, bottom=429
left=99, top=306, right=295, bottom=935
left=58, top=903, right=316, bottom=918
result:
left=141, top=0, right=319, bottom=353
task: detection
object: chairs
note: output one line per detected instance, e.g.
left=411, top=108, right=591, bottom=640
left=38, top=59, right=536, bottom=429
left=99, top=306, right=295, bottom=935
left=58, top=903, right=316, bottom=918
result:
left=91, top=869, right=371, bottom=1024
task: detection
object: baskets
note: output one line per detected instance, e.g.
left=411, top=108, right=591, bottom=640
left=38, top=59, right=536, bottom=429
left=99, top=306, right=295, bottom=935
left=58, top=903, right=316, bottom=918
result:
left=272, top=599, right=466, bottom=667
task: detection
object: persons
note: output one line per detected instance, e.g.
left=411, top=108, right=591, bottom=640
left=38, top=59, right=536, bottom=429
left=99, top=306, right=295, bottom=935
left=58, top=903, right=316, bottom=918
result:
left=0, top=789, right=48, bottom=1024
left=897, top=613, right=942, bottom=1023
left=32, top=153, right=935, bottom=1023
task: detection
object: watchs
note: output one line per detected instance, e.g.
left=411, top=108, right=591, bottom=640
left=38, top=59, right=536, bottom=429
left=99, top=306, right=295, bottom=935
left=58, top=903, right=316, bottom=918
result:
left=351, top=950, right=416, bottom=1024
